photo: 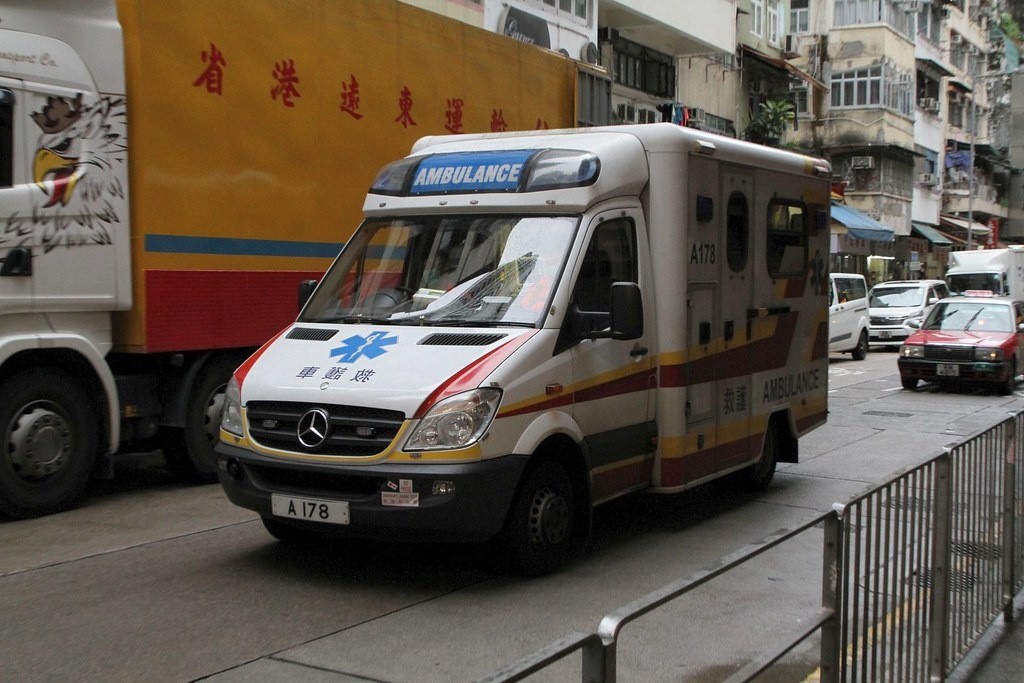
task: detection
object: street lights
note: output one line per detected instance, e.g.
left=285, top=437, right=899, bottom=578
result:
left=966, top=66, right=1024, bottom=251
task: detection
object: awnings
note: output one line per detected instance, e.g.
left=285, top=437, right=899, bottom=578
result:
left=940, top=213, right=993, bottom=236
left=830, top=202, right=896, bottom=245
left=911, top=221, right=954, bottom=247
left=737, top=42, right=832, bottom=94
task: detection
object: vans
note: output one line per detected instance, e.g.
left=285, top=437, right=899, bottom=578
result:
left=826, top=272, right=871, bottom=360
left=867, top=278, right=950, bottom=352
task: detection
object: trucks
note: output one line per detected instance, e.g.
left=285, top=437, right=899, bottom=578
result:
left=943, top=244, right=1024, bottom=319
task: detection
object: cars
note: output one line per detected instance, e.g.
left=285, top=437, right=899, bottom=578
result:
left=898, top=289, right=1024, bottom=396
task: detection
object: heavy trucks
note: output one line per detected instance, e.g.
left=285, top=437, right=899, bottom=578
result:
left=0, top=1, right=617, bottom=523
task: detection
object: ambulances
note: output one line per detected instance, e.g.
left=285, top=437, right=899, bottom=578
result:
left=216, top=121, right=834, bottom=579
